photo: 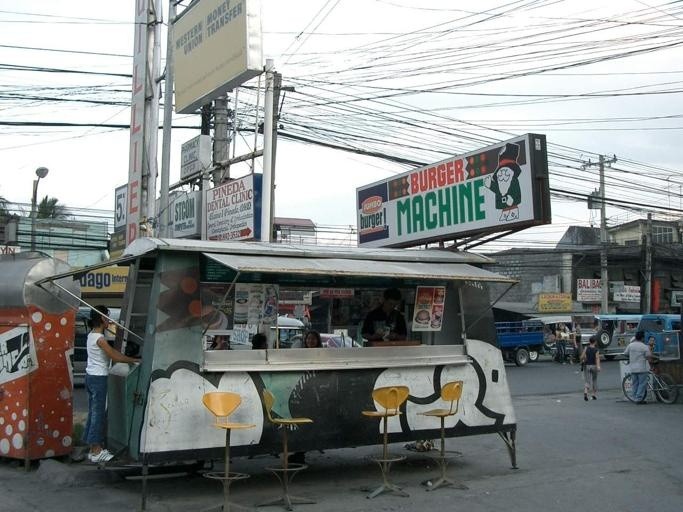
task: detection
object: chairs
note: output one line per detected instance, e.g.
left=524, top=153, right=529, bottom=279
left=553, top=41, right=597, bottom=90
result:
left=416, top=380, right=469, bottom=491
left=256, top=388, right=316, bottom=511
left=202, top=391, right=255, bottom=512
left=362, top=385, right=409, bottom=498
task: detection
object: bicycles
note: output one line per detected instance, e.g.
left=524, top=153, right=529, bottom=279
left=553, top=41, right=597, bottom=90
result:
left=621, top=368, right=682, bottom=404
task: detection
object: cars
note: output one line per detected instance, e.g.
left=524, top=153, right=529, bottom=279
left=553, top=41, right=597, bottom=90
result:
left=271, top=316, right=361, bottom=348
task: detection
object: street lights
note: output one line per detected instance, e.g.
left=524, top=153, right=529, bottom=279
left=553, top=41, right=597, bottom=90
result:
left=29, top=167, right=49, bottom=251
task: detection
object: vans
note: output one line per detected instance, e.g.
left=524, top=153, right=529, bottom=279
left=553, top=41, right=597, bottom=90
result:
left=493, top=313, right=682, bottom=367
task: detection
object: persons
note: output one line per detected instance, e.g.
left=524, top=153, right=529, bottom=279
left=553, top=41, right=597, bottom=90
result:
left=83, top=304, right=141, bottom=463
left=556, top=324, right=658, bottom=404
left=304, top=331, right=321, bottom=348
left=362, top=288, right=406, bottom=341
left=206, top=335, right=230, bottom=350
left=251, top=334, right=268, bottom=350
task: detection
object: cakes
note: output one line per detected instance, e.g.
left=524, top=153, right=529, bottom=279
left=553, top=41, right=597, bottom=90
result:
left=415, top=310, right=430, bottom=325
left=432, top=320, right=441, bottom=329
left=203, top=306, right=228, bottom=330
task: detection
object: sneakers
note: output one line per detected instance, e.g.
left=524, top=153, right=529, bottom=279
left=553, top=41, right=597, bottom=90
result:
left=584, top=392, right=596, bottom=401
left=88, top=449, right=113, bottom=462
left=636, top=401, right=646, bottom=404
